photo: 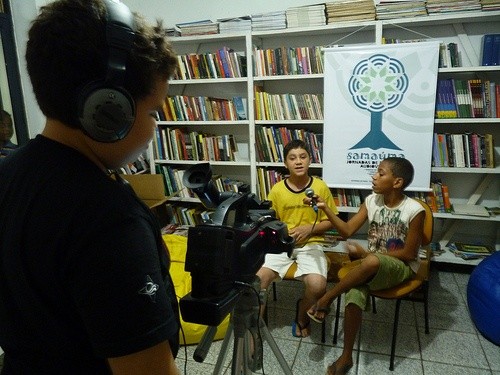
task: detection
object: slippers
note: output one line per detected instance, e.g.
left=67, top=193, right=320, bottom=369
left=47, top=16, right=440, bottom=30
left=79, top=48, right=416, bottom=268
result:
left=327, top=361, right=353, bottom=375
left=306, top=303, right=331, bottom=323
left=292, top=298, right=310, bottom=337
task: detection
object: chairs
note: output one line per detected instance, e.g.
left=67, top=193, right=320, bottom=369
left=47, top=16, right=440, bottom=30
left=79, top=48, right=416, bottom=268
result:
left=331, top=201, right=433, bottom=370
left=266, top=262, right=326, bottom=343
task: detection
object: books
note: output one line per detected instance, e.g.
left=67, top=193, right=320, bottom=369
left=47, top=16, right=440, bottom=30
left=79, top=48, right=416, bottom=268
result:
left=106, top=0, right=500, bottom=261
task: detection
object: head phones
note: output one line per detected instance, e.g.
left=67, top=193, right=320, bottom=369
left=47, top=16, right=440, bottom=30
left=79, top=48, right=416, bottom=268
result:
left=78, top=0, right=138, bottom=144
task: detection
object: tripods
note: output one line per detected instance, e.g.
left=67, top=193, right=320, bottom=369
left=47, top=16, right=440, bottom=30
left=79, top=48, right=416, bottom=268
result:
left=193, top=278, right=293, bottom=375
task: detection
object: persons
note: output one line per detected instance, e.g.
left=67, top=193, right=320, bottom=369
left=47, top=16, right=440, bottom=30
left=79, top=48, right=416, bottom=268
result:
left=253, top=140, right=345, bottom=338
left=0, top=1, right=184, bottom=375
left=301, top=158, right=424, bottom=375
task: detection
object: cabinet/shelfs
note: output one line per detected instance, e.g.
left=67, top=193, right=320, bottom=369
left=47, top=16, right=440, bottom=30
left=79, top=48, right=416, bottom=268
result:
left=163, top=11, right=500, bottom=266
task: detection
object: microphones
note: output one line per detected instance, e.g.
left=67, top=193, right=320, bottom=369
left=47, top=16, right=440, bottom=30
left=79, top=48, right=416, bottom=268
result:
left=305, top=188, right=318, bottom=214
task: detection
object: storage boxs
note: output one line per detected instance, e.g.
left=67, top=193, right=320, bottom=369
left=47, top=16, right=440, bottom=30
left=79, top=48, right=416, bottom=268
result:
left=113, top=170, right=186, bottom=221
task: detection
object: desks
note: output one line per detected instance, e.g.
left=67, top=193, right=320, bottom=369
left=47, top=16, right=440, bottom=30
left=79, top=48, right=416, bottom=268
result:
left=164, top=231, right=234, bottom=343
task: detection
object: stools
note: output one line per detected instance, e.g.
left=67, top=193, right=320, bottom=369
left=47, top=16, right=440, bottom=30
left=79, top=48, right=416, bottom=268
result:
left=467, top=239, right=500, bottom=351
